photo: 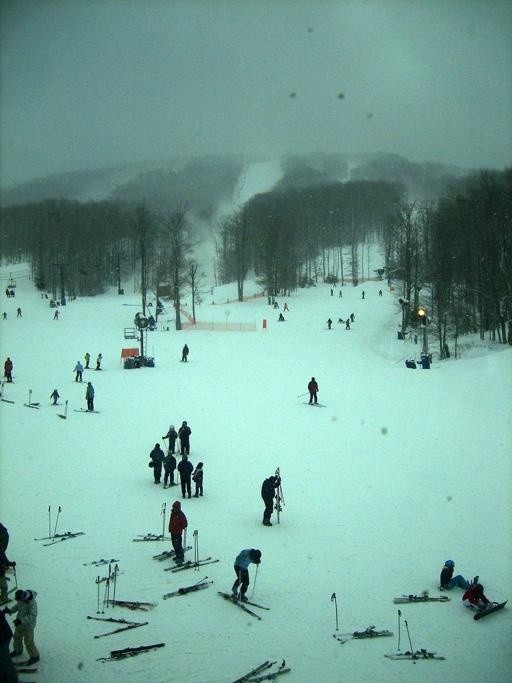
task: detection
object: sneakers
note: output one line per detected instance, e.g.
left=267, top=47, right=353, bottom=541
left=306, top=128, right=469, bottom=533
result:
left=10, top=650, right=40, bottom=665
left=172, top=521, right=272, bottom=601
left=153, top=480, right=204, bottom=499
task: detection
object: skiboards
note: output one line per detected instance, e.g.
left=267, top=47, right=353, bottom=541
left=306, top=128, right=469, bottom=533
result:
left=333, top=626, right=393, bottom=644
left=394, top=596, right=448, bottom=603
left=87, top=616, right=148, bottom=638
left=233, top=661, right=291, bottom=683
left=152, top=546, right=192, bottom=561
left=218, top=592, right=270, bottom=621
left=133, top=534, right=171, bottom=541
left=73, top=408, right=100, bottom=413
left=96, top=643, right=165, bottom=663
left=83, top=559, right=119, bottom=566
left=164, top=557, right=219, bottom=573
left=34, top=532, right=85, bottom=546
left=304, top=403, right=326, bottom=408
left=107, top=600, right=153, bottom=611
left=385, top=653, right=445, bottom=660
left=163, top=576, right=213, bottom=600
left=14, top=661, right=37, bottom=674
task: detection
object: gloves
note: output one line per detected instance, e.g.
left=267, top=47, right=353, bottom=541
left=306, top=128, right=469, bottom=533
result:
left=12, top=618, right=22, bottom=627
left=2, top=605, right=13, bottom=616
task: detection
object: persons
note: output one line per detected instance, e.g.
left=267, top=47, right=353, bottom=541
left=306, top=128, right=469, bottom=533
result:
left=261, top=475, right=281, bottom=526
left=5, top=361, right=13, bottom=382
left=169, top=500, right=188, bottom=563
left=178, top=420, right=192, bottom=455
left=50, top=388, right=60, bottom=404
left=96, top=353, right=102, bottom=369
left=346, top=318, right=350, bottom=329
left=350, top=313, right=355, bottom=321
left=150, top=442, right=164, bottom=484
left=182, top=343, right=189, bottom=361
left=192, top=461, right=204, bottom=498
left=462, top=583, right=491, bottom=611
left=178, top=454, right=193, bottom=498
left=439, top=559, right=478, bottom=591
left=17, top=307, right=23, bottom=317
left=326, top=318, right=332, bottom=328
left=4, top=357, right=12, bottom=377
left=4, top=589, right=40, bottom=667
left=161, top=423, right=177, bottom=454
left=3, top=311, right=8, bottom=319
left=284, top=302, right=289, bottom=311
left=53, top=309, right=60, bottom=319
left=84, top=352, right=90, bottom=368
left=73, top=360, right=84, bottom=382
left=162, top=448, right=176, bottom=488
left=308, top=376, right=319, bottom=405
left=86, top=381, right=95, bottom=412
left=232, top=549, right=262, bottom=602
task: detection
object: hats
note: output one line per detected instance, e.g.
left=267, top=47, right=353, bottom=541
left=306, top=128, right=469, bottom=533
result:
left=14, top=589, right=28, bottom=602
left=154, top=421, right=203, bottom=469
left=172, top=501, right=180, bottom=509
left=444, top=559, right=455, bottom=567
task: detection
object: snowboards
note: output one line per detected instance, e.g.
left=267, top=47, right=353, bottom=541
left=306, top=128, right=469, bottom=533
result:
left=474, top=601, right=508, bottom=620
